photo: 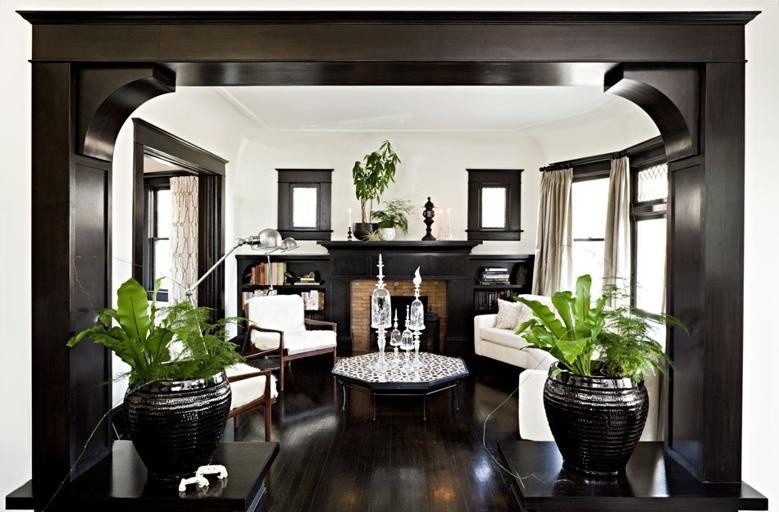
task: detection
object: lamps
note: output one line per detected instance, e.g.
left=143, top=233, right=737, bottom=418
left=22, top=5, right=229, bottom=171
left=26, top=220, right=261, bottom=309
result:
left=184, top=228, right=299, bottom=354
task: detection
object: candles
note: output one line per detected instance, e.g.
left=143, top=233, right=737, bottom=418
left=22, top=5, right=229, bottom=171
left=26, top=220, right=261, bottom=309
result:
left=348, top=209, right=351, bottom=227
left=446, top=208, right=451, bottom=225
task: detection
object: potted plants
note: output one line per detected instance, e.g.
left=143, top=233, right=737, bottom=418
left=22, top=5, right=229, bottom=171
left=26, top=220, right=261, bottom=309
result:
left=515, top=268, right=690, bottom=488
left=351, top=139, right=402, bottom=240
left=66, top=278, right=257, bottom=482
left=370, top=198, right=416, bottom=240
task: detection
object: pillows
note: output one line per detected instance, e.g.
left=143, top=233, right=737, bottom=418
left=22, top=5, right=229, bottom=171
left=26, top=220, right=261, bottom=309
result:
left=493, top=297, right=519, bottom=328
left=516, top=305, right=554, bottom=332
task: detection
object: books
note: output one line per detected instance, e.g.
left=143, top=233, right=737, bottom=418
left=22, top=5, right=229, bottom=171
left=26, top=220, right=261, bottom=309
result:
left=474, top=266, right=528, bottom=311
left=242, top=262, right=326, bottom=330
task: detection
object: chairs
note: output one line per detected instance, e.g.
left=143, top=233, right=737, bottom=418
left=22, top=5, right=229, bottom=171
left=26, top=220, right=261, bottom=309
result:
left=219, top=363, right=279, bottom=443
left=241, top=295, right=339, bottom=408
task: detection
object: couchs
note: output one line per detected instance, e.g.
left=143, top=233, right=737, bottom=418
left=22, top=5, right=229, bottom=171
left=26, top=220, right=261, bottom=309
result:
left=473, top=293, right=576, bottom=393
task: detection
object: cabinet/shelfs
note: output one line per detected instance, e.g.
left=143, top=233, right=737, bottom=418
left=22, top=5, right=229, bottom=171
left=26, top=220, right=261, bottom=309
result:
left=497, top=440, right=768, bottom=510
left=5, top=440, right=282, bottom=512
left=468, top=254, right=536, bottom=376
left=235, top=255, right=330, bottom=335
